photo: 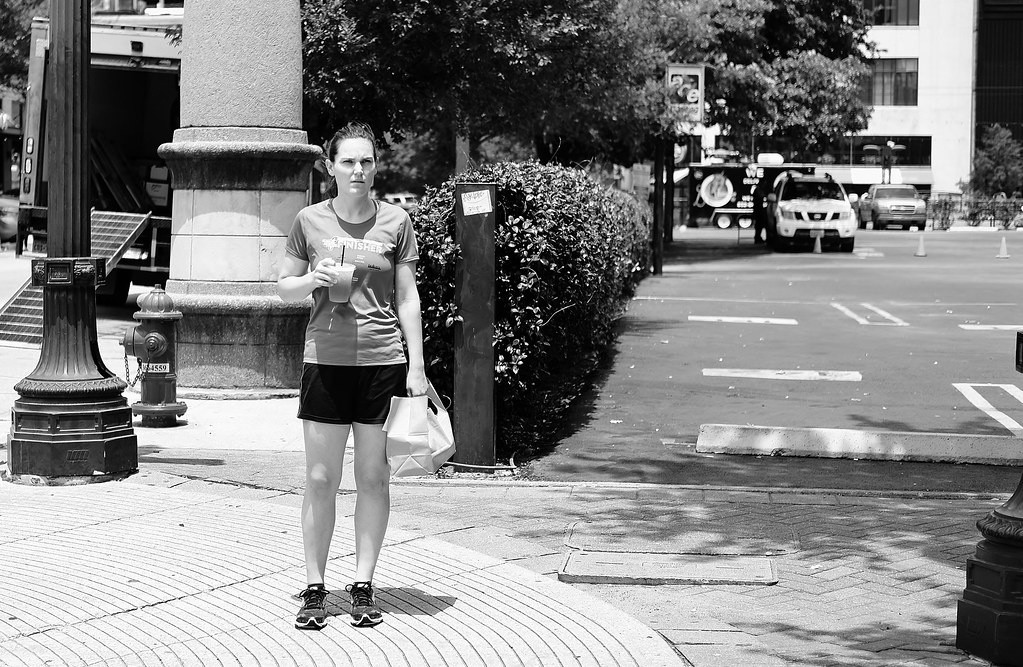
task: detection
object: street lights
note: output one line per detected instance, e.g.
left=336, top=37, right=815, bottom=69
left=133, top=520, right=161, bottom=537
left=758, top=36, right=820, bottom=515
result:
left=862, top=141, right=906, bottom=184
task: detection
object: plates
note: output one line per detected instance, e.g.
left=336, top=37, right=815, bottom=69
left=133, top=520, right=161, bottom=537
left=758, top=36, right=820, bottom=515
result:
left=700, top=174, right=734, bottom=207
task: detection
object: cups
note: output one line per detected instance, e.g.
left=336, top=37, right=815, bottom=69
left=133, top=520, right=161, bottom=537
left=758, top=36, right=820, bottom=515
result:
left=328, top=263, right=356, bottom=303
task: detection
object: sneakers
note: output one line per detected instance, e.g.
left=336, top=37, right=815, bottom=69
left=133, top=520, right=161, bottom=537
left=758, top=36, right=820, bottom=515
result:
left=345, top=581, right=383, bottom=626
left=295, top=585, right=329, bottom=629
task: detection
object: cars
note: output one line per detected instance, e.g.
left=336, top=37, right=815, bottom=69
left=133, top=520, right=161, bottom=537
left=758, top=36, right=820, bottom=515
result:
left=860, top=182, right=927, bottom=231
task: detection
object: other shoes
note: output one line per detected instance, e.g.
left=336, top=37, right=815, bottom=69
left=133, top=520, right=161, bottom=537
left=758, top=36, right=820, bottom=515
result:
left=754, top=236, right=765, bottom=244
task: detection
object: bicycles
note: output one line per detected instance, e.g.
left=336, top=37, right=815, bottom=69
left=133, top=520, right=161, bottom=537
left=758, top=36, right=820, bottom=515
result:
left=932, top=198, right=1023, bottom=231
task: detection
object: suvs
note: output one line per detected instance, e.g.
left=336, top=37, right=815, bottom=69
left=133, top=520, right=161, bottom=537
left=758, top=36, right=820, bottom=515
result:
left=764, top=170, right=860, bottom=256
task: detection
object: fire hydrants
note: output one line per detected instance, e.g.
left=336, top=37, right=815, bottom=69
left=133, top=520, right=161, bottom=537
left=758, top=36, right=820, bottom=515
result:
left=118, top=284, right=189, bottom=428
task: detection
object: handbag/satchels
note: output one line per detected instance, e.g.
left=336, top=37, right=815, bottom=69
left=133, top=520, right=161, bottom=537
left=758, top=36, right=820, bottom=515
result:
left=382, top=378, right=457, bottom=476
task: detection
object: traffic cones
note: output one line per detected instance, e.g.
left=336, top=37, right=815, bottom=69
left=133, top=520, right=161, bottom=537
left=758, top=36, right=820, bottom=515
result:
left=995, top=236, right=1010, bottom=259
left=812, top=234, right=822, bottom=254
left=914, top=235, right=927, bottom=257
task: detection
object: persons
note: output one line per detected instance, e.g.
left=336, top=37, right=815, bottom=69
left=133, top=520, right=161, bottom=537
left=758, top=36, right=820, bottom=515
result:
left=278, top=125, right=429, bottom=631
left=752, top=176, right=771, bottom=244
